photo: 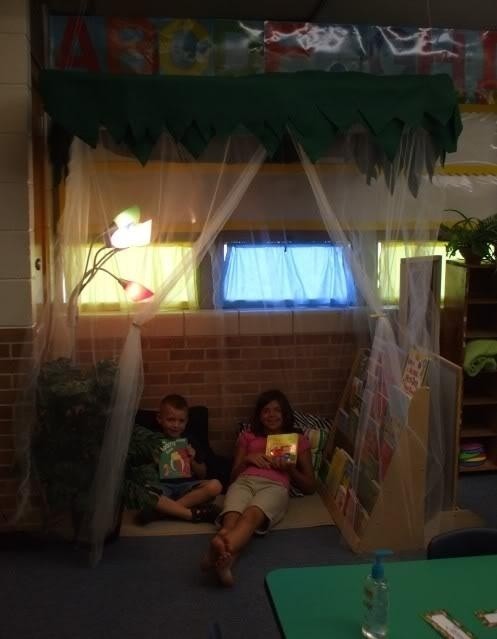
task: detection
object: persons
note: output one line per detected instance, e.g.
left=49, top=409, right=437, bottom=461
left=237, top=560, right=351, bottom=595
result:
left=144, top=392, right=224, bottom=523
left=199, top=388, right=317, bottom=588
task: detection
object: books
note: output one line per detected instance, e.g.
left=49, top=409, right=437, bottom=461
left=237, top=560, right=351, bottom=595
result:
left=158, top=437, right=192, bottom=481
left=265, top=432, right=299, bottom=465
left=318, top=334, right=432, bottom=539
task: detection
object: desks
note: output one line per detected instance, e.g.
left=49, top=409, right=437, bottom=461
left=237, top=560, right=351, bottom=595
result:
left=264, top=555, right=497, bottom=639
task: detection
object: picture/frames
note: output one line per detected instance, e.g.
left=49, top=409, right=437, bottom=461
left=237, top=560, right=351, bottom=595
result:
left=439, top=356, right=463, bottom=511
left=398, top=255, right=440, bottom=356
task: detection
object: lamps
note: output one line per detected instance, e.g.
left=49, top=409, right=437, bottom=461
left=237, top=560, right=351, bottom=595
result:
left=66, top=205, right=156, bottom=329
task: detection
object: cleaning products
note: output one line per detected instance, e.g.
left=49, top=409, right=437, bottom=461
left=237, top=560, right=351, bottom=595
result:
left=362, top=548, right=397, bottom=639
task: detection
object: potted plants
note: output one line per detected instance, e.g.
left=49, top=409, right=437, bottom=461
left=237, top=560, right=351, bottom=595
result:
left=32, top=357, right=170, bottom=545
left=444, top=209, right=497, bottom=264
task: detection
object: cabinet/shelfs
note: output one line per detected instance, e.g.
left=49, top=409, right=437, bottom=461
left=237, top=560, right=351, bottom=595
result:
left=315, top=348, right=430, bottom=556
left=442, top=259, right=497, bottom=474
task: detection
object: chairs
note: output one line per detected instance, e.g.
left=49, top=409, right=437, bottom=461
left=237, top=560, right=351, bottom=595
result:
left=428, top=527, right=497, bottom=558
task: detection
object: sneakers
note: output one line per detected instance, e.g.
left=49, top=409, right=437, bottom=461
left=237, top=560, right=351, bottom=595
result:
left=190, top=503, right=226, bottom=523
left=135, top=509, right=161, bottom=526
